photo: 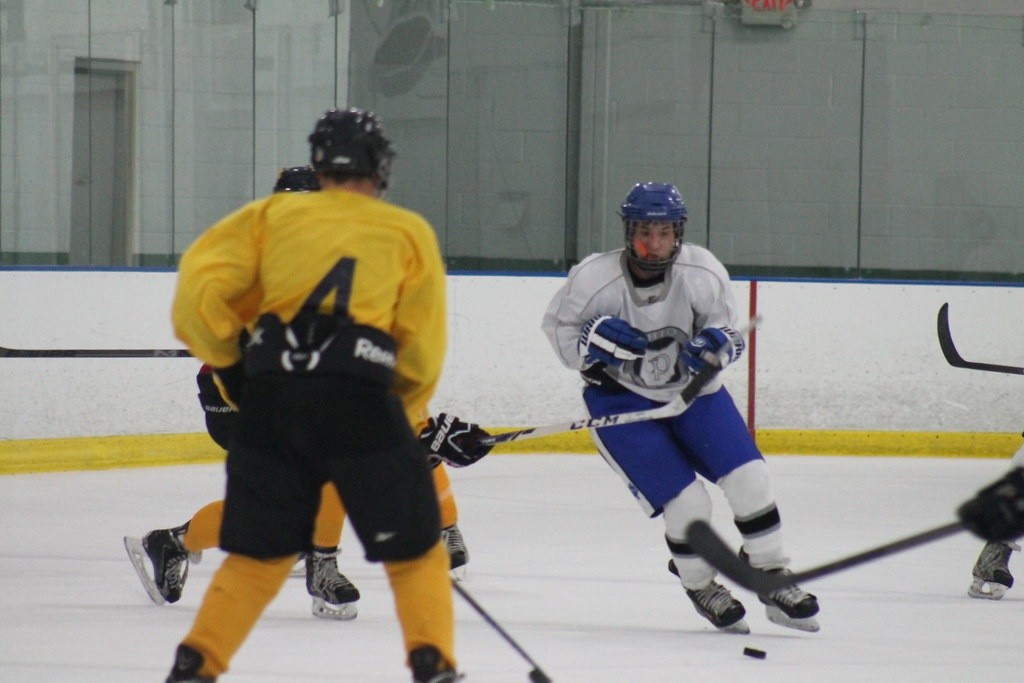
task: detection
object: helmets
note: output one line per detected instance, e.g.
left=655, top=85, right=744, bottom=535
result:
left=308, top=109, right=393, bottom=188
left=274, top=166, right=320, bottom=192
left=621, top=183, right=689, bottom=235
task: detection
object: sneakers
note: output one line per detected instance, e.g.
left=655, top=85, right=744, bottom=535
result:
left=686, top=582, right=746, bottom=632
left=967, top=540, right=1014, bottom=599
left=440, top=526, right=467, bottom=581
left=124, top=523, right=203, bottom=606
left=757, top=568, right=821, bottom=636
left=306, top=543, right=361, bottom=620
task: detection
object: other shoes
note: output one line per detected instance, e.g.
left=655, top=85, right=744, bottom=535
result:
left=165, top=643, right=221, bottom=683
left=410, top=644, right=462, bottom=683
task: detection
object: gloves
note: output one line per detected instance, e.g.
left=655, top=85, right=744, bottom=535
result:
left=196, top=361, right=245, bottom=449
left=681, top=325, right=746, bottom=377
left=958, top=465, right=1024, bottom=540
left=420, top=411, right=495, bottom=467
left=578, top=313, right=647, bottom=367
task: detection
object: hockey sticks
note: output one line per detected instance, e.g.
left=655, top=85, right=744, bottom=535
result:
left=683, top=518, right=970, bottom=595
left=451, top=577, right=554, bottom=683
left=478, top=362, right=724, bottom=447
left=937, top=302, right=1023, bottom=375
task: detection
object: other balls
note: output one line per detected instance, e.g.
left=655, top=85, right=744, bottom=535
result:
left=743, top=647, right=767, bottom=659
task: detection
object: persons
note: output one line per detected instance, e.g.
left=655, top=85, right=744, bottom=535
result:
left=542, top=181, right=821, bottom=632
left=959, top=444, right=1024, bottom=600
left=124, top=106, right=494, bottom=683
